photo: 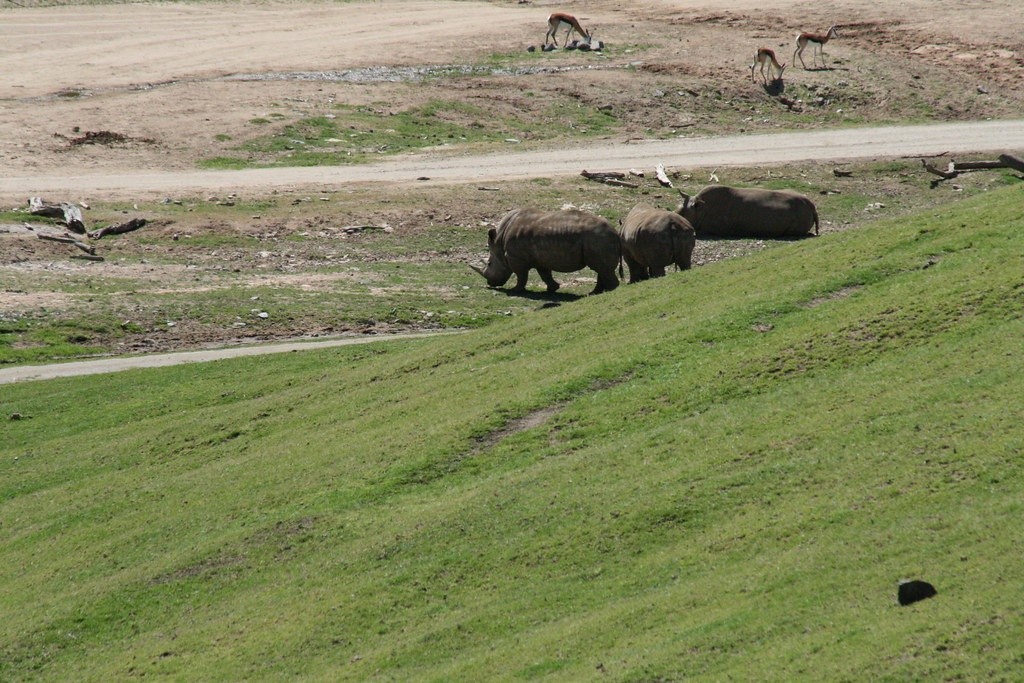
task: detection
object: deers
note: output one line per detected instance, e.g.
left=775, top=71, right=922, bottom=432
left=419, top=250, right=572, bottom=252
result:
left=751, top=48, right=788, bottom=86
left=546, top=13, right=593, bottom=47
left=792, top=24, right=839, bottom=68
